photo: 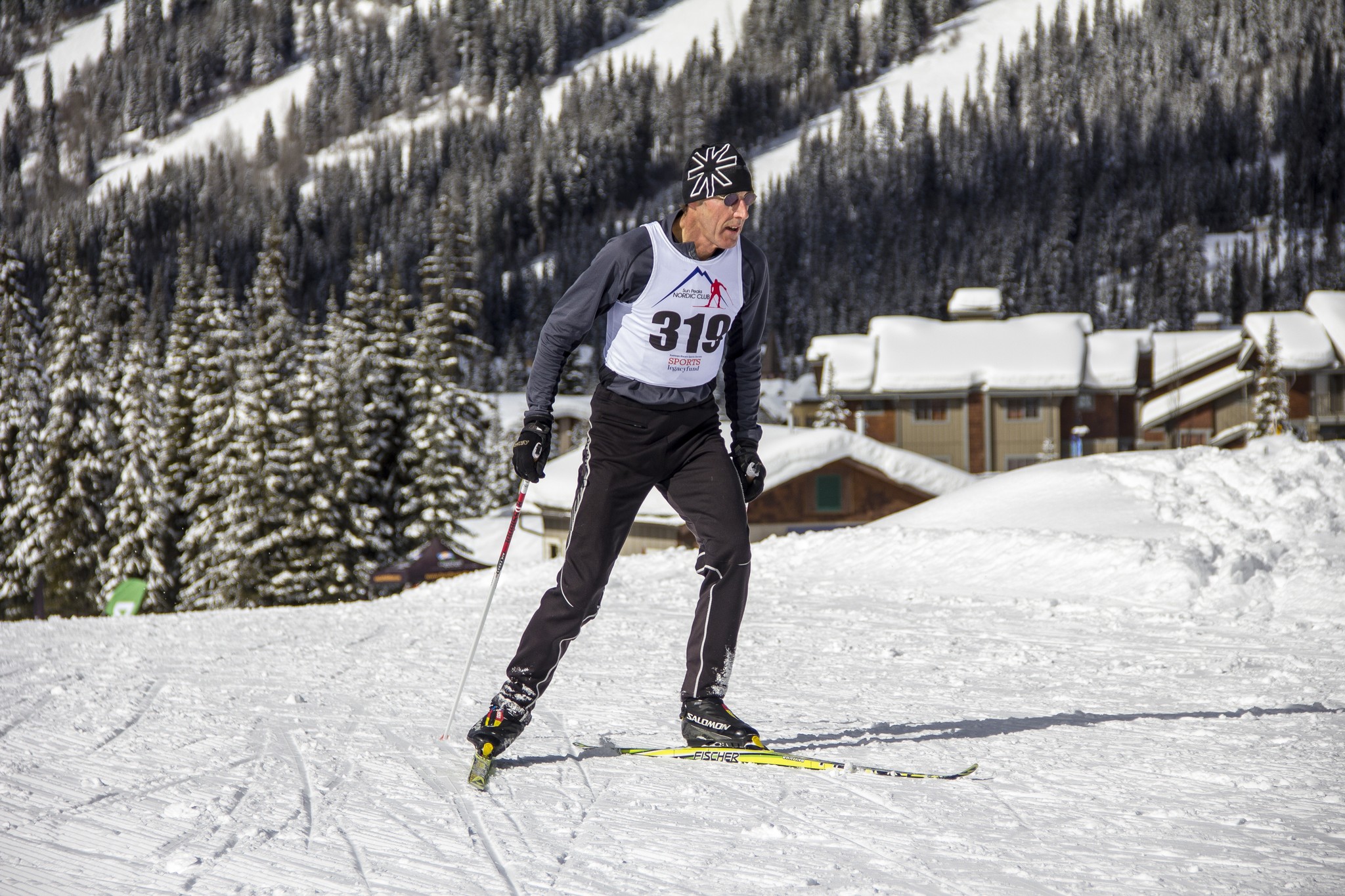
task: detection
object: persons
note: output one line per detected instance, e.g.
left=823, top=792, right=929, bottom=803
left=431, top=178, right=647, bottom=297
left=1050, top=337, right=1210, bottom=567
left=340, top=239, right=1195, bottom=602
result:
left=464, top=141, right=767, bottom=754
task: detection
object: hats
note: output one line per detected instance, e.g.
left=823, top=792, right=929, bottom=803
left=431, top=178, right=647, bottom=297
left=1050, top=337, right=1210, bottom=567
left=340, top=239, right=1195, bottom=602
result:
left=682, top=142, right=753, bottom=204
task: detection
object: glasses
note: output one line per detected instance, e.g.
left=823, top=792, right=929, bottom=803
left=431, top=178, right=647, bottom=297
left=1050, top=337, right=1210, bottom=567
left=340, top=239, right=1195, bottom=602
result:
left=717, top=193, right=757, bottom=207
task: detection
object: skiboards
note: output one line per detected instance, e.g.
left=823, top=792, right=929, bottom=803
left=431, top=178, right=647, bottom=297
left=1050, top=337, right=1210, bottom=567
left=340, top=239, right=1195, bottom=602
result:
left=466, top=741, right=979, bottom=792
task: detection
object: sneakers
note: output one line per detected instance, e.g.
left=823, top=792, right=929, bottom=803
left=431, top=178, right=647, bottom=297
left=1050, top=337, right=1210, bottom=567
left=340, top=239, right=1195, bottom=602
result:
left=679, top=696, right=760, bottom=748
left=467, top=703, right=526, bottom=757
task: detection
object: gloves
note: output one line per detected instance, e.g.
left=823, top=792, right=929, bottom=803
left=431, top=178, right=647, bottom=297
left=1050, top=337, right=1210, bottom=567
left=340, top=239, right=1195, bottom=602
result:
left=730, top=439, right=767, bottom=503
left=512, top=413, right=555, bottom=483
